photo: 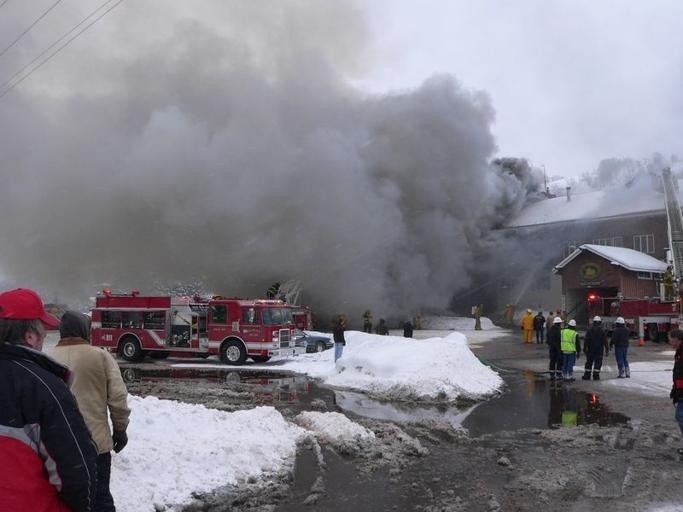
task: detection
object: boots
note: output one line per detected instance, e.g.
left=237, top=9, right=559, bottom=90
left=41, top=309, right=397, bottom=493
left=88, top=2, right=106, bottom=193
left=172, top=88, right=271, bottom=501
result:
left=549, top=370, right=555, bottom=381
left=581, top=369, right=591, bottom=381
left=557, top=371, right=564, bottom=380
left=593, top=369, right=601, bottom=381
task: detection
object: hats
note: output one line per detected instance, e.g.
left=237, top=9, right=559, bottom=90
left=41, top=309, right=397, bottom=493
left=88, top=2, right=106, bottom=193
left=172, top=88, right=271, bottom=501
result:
left=2, top=286, right=63, bottom=327
left=553, top=316, right=563, bottom=324
left=616, top=317, right=625, bottom=325
left=568, top=319, right=577, bottom=326
left=592, top=315, right=602, bottom=322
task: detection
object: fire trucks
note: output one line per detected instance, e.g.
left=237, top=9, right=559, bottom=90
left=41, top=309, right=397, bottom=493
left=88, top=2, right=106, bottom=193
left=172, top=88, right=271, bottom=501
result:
left=117, top=366, right=302, bottom=412
left=583, top=163, right=682, bottom=347
left=289, top=302, right=312, bottom=331
left=85, top=288, right=297, bottom=365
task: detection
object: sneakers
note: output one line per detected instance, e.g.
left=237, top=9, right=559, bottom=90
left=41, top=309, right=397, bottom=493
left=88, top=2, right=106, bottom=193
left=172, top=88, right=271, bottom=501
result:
left=627, top=375, right=631, bottom=378
left=565, top=377, right=577, bottom=381
left=616, top=375, right=626, bottom=379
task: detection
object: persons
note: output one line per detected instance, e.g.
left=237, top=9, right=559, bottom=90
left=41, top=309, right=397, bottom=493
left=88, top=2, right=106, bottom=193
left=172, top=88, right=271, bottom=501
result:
left=266, top=282, right=280, bottom=300
left=608, top=317, right=630, bottom=378
left=374, top=319, right=388, bottom=336
left=559, top=320, right=580, bottom=381
left=42, top=310, right=131, bottom=511
left=402, top=321, right=412, bottom=338
left=546, top=317, right=562, bottom=379
left=581, top=316, right=608, bottom=381
left=499, top=304, right=512, bottom=326
left=533, top=312, right=545, bottom=344
left=362, top=309, right=372, bottom=334
left=520, top=309, right=533, bottom=343
left=667, top=330, right=682, bottom=454
left=473, top=303, right=483, bottom=330
left=332, top=316, right=345, bottom=372
left=0, top=289, right=97, bottom=511
left=545, top=311, right=555, bottom=344
left=552, top=311, right=563, bottom=322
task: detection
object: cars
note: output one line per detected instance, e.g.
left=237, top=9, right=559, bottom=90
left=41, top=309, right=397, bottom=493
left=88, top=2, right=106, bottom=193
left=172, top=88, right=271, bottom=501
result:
left=294, top=328, right=335, bottom=355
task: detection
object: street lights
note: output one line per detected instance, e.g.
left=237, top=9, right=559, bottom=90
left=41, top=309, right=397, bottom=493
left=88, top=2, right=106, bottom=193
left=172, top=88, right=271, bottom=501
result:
left=539, top=163, right=545, bottom=190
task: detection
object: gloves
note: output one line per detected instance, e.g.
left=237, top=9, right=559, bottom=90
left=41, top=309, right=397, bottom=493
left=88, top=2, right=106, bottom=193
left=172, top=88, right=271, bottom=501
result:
left=112, top=428, right=129, bottom=452
